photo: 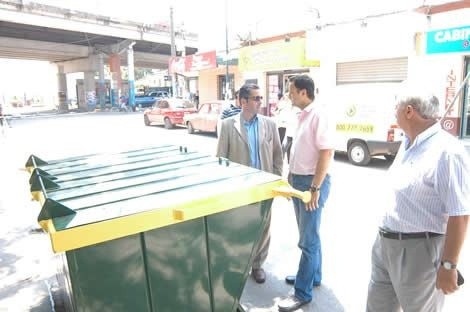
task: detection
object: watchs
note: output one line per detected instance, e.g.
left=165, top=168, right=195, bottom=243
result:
left=441, top=260, right=456, bottom=270
left=309, top=185, right=320, bottom=192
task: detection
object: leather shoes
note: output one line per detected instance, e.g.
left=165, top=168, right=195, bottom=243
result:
left=253, top=269, right=266, bottom=283
left=278, top=294, right=312, bottom=312
left=286, top=276, right=295, bottom=283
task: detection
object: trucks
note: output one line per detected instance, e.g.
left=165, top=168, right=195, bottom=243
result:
left=275, top=83, right=405, bottom=167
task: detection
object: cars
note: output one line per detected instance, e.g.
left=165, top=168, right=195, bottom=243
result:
left=184, top=100, right=235, bottom=134
left=144, top=99, right=196, bottom=129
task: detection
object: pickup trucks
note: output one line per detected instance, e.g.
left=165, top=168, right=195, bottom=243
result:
left=135, top=91, right=168, bottom=109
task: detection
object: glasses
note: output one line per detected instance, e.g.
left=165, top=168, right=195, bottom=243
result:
left=248, top=96, right=263, bottom=101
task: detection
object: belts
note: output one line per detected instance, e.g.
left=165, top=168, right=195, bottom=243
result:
left=379, top=229, right=442, bottom=240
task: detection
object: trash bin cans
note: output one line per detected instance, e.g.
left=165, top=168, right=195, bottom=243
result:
left=25, top=141, right=313, bottom=311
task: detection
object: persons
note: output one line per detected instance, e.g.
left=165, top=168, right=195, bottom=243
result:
left=278, top=74, right=335, bottom=311
left=366, top=79, right=470, bottom=312
left=215, top=80, right=291, bottom=284
